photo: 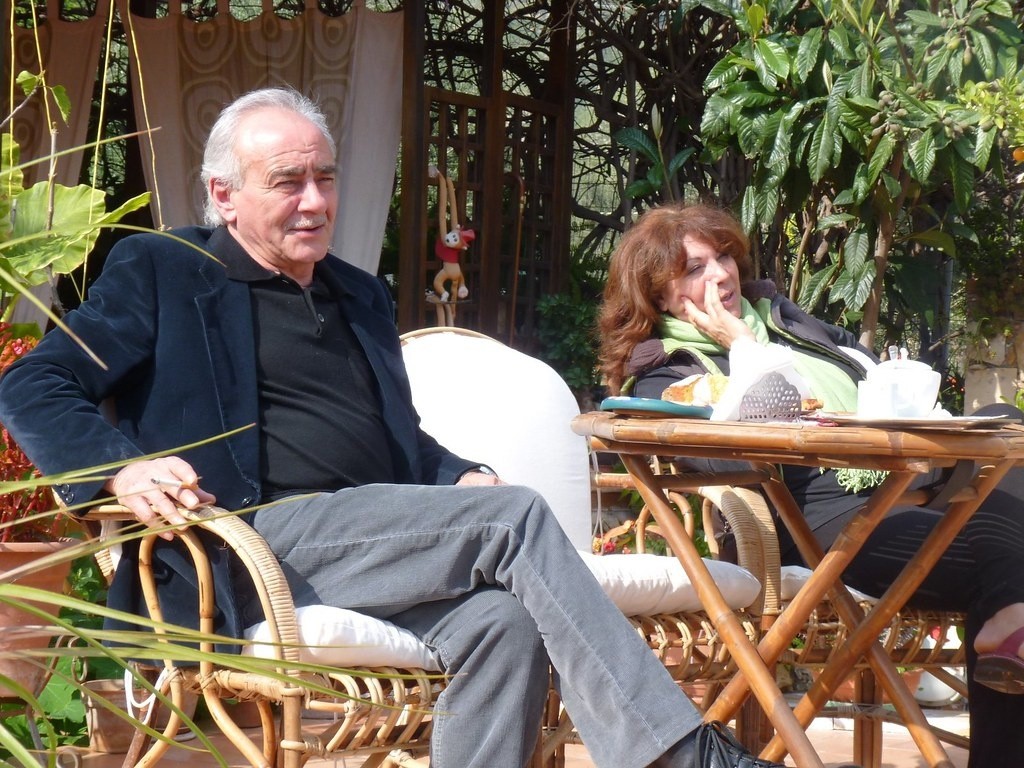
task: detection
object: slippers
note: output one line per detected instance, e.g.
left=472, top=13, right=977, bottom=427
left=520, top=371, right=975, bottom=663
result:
left=973, top=626, right=1024, bottom=695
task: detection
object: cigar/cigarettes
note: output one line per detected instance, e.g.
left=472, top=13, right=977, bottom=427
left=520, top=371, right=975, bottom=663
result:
left=150, top=476, right=199, bottom=490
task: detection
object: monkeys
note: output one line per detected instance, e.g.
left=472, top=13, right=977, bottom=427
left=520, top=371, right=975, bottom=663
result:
left=429, top=167, right=475, bottom=303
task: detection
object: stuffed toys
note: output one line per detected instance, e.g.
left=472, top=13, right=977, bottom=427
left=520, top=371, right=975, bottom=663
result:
left=431, top=170, right=474, bottom=302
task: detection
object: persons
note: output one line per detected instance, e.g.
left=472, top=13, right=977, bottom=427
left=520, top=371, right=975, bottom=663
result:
left=595, top=203, right=1023, bottom=767
left=0, top=88, right=782, bottom=768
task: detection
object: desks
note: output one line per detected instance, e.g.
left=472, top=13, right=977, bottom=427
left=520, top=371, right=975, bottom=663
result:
left=575, top=410, right=1024, bottom=768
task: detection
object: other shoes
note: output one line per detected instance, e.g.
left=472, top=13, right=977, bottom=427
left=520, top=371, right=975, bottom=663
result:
left=648, top=719, right=786, bottom=768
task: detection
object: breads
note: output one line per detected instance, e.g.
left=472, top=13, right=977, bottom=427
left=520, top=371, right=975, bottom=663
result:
left=800, top=398, right=824, bottom=410
left=661, top=374, right=730, bottom=405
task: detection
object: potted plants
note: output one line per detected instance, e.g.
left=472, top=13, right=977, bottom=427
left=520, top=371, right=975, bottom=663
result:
left=0, top=72, right=151, bottom=699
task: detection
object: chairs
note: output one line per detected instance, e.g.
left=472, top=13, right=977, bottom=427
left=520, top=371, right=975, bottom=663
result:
left=53, top=326, right=968, bottom=768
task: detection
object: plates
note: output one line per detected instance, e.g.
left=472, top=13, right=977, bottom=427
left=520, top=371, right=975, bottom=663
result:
left=817, top=407, right=1022, bottom=428
